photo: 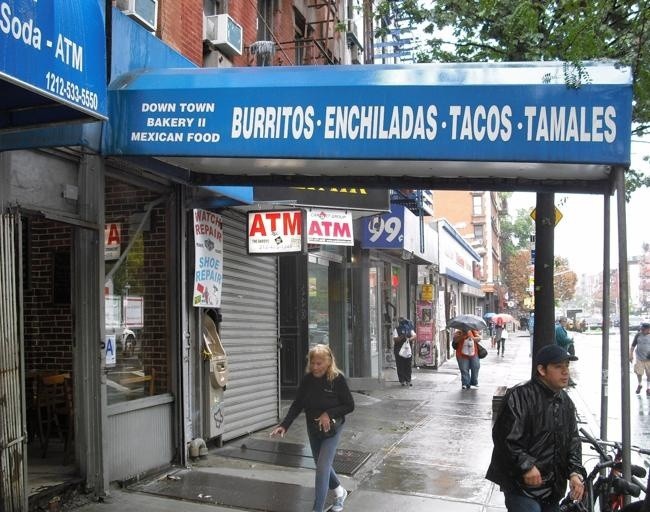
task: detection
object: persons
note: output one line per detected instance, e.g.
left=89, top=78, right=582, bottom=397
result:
left=484, top=344, right=587, bottom=511
left=453, top=328, right=482, bottom=390
left=496, top=317, right=506, bottom=357
left=629, top=323, right=650, bottom=396
left=488, top=317, right=496, bottom=348
left=267, top=343, right=354, bottom=512
left=529, top=313, right=534, bottom=358
left=392, top=317, right=417, bottom=387
left=555, top=316, right=577, bottom=387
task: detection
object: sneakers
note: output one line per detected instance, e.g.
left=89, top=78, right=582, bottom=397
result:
left=462, top=384, right=480, bottom=389
left=402, top=381, right=413, bottom=386
left=331, top=489, right=347, bottom=512
left=636, top=386, right=650, bottom=396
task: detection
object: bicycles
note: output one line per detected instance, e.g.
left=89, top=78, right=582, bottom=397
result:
left=560, top=428, right=649, bottom=512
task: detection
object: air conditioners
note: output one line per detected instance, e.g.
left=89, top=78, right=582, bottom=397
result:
left=206, top=14, right=245, bottom=57
left=116, top=0, right=160, bottom=32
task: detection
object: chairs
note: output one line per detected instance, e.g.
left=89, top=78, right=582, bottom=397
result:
left=25, top=371, right=74, bottom=462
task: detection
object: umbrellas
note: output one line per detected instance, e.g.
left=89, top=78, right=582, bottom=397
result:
left=492, top=313, right=513, bottom=323
left=396, top=319, right=415, bottom=341
left=447, top=313, right=487, bottom=335
left=484, top=312, right=496, bottom=318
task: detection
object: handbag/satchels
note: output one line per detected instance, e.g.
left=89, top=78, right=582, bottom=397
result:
left=399, top=338, right=412, bottom=359
left=452, top=341, right=457, bottom=349
left=568, top=344, right=575, bottom=355
left=501, top=329, right=508, bottom=340
left=477, top=344, right=487, bottom=358
left=462, top=337, right=475, bottom=357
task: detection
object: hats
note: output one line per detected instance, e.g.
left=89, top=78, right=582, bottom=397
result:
left=637, top=323, right=650, bottom=330
left=536, top=345, right=578, bottom=363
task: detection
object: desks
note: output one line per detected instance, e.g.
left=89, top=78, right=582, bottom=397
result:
left=108, top=372, right=153, bottom=402
